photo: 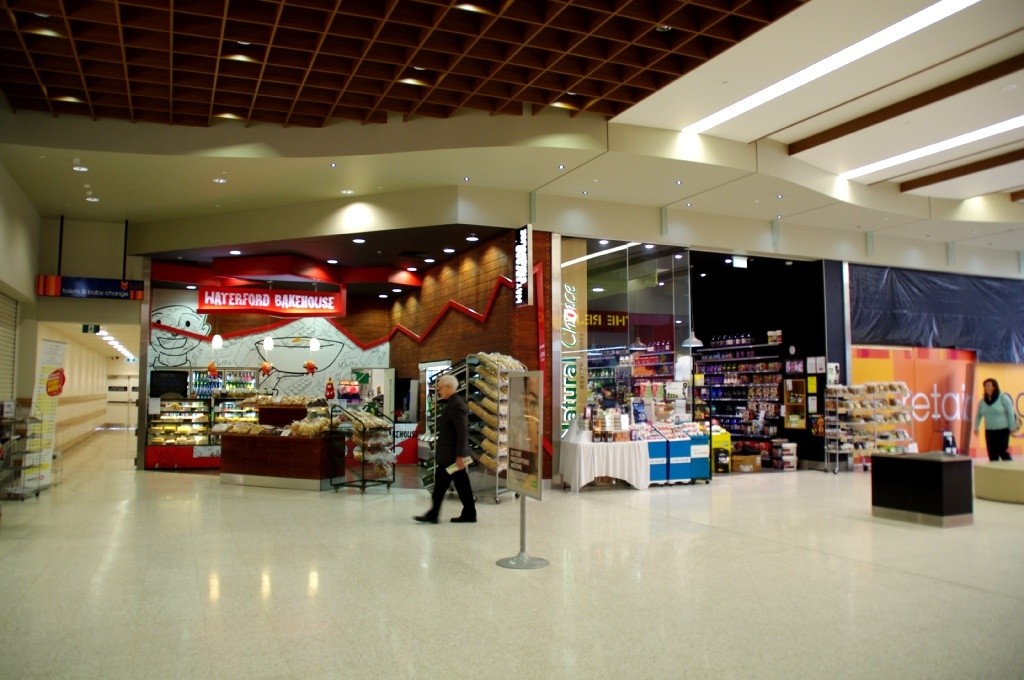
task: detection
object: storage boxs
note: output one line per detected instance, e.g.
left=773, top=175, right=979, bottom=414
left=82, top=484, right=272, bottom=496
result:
left=731, top=455, right=762, bottom=472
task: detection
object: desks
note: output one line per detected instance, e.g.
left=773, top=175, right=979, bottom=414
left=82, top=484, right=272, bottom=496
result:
left=559, top=440, right=650, bottom=491
left=711, top=432, right=731, bottom=473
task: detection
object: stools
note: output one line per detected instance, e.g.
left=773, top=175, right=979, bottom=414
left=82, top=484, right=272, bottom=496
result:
left=975, top=461, right=1024, bottom=503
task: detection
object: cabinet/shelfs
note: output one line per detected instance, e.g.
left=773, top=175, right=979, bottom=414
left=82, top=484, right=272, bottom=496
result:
left=825, top=381, right=912, bottom=474
left=149, top=367, right=259, bottom=447
left=330, top=405, right=395, bottom=493
left=588, top=343, right=806, bottom=439
left=426, top=353, right=528, bottom=503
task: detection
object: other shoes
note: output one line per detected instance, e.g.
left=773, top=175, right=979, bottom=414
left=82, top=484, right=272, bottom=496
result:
left=450, top=517, right=476, bottom=523
left=413, top=515, right=438, bottom=524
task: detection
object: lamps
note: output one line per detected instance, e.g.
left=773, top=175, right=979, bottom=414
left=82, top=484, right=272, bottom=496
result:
left=629, top=302, right=647, bottom=350
left=681, top=250, right=703, bottom=347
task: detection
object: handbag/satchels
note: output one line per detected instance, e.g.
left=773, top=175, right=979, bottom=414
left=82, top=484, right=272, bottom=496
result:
left=1002, top=392, right=1022, bottom=432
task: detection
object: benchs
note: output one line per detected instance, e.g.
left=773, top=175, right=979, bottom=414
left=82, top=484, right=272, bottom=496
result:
left=871, top=453, right=973, bottom=517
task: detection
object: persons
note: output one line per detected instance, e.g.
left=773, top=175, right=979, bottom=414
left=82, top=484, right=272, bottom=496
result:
left=601, top=382, right=615, bottom=410
left=974, top=378, right=1016, bottom=463
left=414, top=375, right=477, bottom=523
